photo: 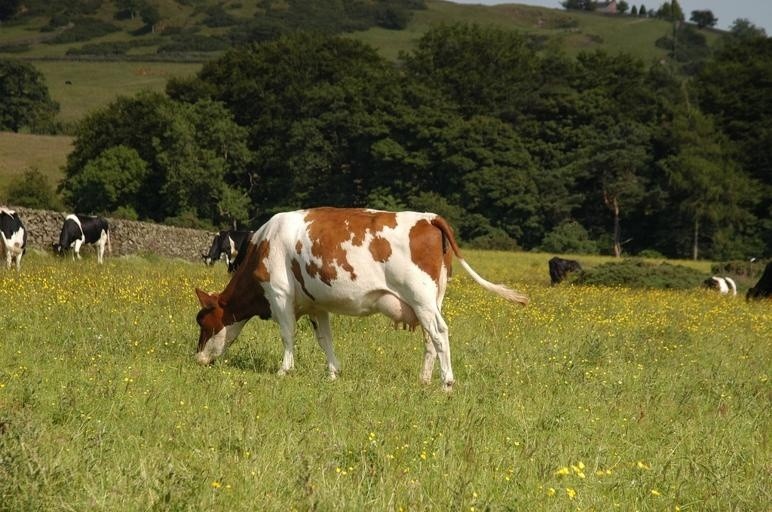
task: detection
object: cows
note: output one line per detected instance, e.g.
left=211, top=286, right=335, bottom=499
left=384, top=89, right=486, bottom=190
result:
left=0, top=207, right=28, bottom=271
left=52, top=213, right=114, bottom=265
left=190, top=205, right=529, bottom=393
left=701, top=276, right=737, bottom=297
left=548, top=255, right=584, bottom=287
